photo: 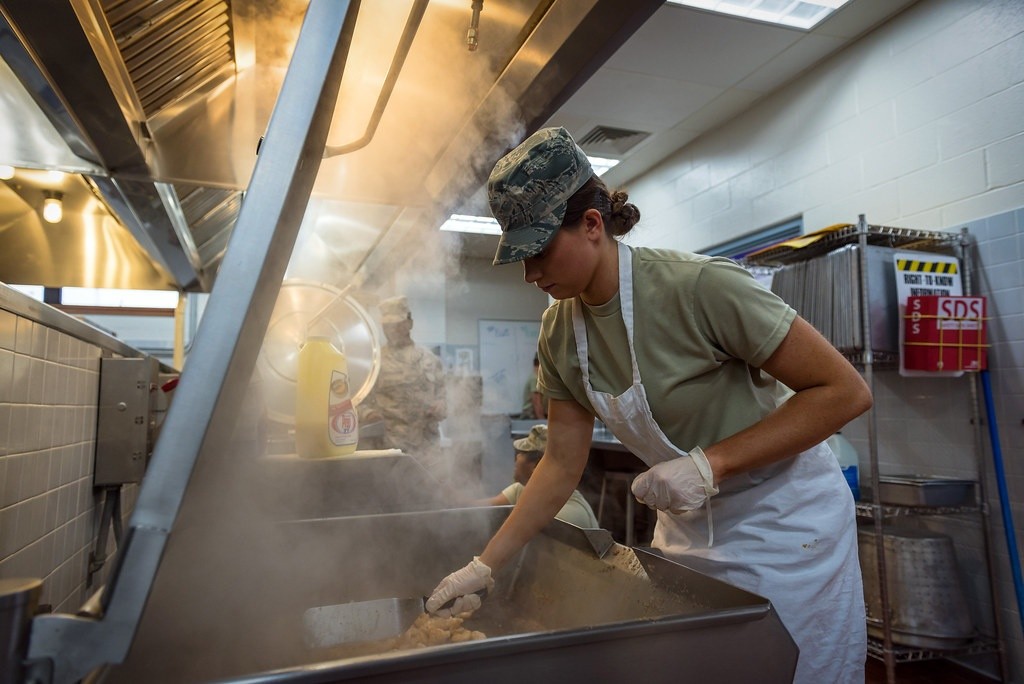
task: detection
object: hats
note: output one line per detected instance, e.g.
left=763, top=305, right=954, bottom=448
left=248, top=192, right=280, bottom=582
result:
left=380, top=296, right=410, bottom=325
left=513, top=425, right=547, bottom=454
left=485, top=126, right=594, bottom=266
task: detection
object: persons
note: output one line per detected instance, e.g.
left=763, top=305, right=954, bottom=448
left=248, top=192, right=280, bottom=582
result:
left=521, top=350, right=552, bottom=420
left=365, top=293, right=454, bottom=450
left=426, top=126, right=877, bottom=684
left=448, top=420, right=602, bottom=535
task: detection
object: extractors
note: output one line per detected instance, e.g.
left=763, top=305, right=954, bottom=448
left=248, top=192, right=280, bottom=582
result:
left=0, top=0, right=667, bottom=290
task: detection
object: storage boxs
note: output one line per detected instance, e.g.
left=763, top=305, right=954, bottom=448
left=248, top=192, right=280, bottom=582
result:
left=877, top=473, right=980, bottom=508
left=854, top=523, right=979, bottom=651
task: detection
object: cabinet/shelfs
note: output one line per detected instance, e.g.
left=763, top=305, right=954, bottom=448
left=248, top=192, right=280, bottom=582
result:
left=738, top=213, right=1010, bottom=684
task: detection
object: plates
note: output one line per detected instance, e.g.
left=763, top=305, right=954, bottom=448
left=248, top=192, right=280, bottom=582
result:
left=762, top=241, right=952, bottom=353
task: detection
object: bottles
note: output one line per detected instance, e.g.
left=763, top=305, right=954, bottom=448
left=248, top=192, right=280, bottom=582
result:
left=294, top=337, right=358, bottom=462
left=828, top=431, right=859, bottom=502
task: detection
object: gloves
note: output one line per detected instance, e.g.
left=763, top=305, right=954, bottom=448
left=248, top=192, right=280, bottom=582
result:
left=425, top=556, right=494, bottom=617
left=631, top=446, right=719, bottom=515
